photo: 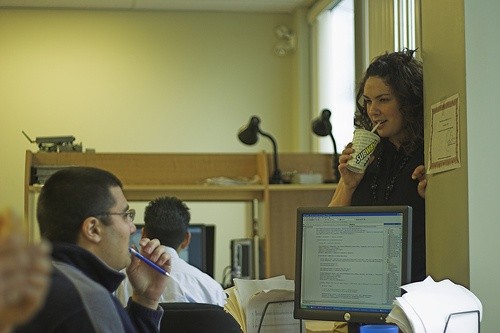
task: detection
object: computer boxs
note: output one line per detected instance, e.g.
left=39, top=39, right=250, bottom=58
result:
left=230, top=240, right=252, bottom=284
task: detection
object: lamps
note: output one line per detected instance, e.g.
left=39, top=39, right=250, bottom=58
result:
left=272, top=24, right=298, bottom=58
left=311, top=108, right=343, bottom=184
left=237, top=115, right=290, bottom=184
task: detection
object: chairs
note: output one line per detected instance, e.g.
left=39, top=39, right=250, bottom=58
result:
left=158, top=302, right=245, bottom=333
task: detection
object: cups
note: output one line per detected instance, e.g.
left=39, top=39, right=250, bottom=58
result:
left=346, top=128, right=381, bottom=174
left=360, top=324, right=399, bottom=333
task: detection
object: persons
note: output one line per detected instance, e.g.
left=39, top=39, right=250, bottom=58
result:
left=110, top=195, right=228, bottom=306
left=327, top=46, right=425, bottom=279
left=0, top=209, right=53, bottom=333
left=16, top=168, right=173, bottom=333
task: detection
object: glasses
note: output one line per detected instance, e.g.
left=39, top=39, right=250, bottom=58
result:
left=100, top=209, right=136, bottom=222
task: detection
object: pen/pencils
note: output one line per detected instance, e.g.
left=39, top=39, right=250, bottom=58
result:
left=129, top=248, right=170, bottom=277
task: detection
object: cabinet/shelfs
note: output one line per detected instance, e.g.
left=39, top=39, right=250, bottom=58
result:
left=22, top=148, right=343, bottom=279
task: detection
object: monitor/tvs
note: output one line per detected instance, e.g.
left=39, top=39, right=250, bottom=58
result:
left=293, top=206, right=413, bottom=332
left=128, top=222, right=207, bottom=282
